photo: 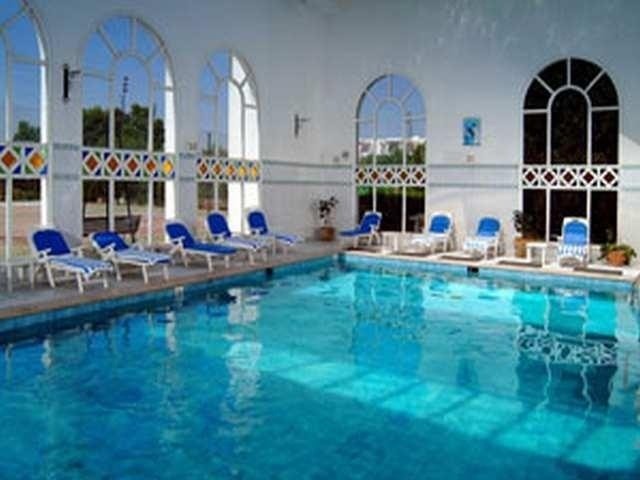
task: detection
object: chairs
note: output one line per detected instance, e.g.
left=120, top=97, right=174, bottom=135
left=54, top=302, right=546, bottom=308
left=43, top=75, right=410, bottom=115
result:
left=88, top=230, right=176, bottom=285
left=204, top=212, right=271, bottom=265
left=405, top=211, right=454, bottom=256
left=246, top=210, right=304, bottom=256
left=339, top=211, right=383, bottom=249
left=556, top=217, right=589, bottom=269
left=27, top=228, right=114, bottom=294
left=464, top=216, right=502, bottom=264
left=163, top=222, right=236, bottom=272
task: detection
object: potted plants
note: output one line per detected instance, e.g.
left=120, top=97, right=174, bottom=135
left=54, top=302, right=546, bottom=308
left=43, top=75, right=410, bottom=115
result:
left=319, top=196, right=339, bottom=241
left=511, top=210, right=536, bottom=257
left=597, top=241, right=637, bottom=267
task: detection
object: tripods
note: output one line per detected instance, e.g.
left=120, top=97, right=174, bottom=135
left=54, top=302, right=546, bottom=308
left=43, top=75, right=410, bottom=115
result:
left=83, top=137, right=136, bottom=242
